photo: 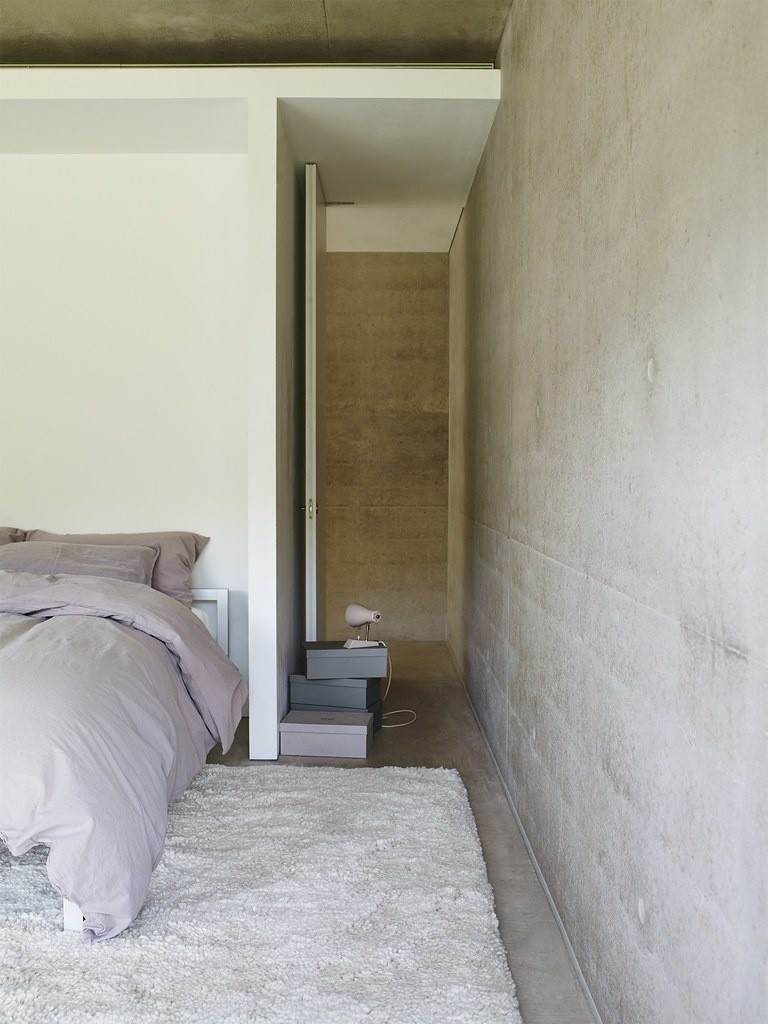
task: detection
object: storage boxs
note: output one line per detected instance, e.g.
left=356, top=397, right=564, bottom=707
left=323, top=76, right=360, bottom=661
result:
left=298, top=640, right=389, bottom=680
left=290, top=669, right=381, bottom=708
left=289, top=701, right=383, bottom=733
left=279, top=710, right=375, bottom=759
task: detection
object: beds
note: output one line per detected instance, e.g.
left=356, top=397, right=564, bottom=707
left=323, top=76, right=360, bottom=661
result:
left=0, top=570, right=249, bottom=941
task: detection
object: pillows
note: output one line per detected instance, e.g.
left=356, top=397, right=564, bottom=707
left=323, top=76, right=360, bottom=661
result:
left=0, top=540, right=161, bottom=588
left=27, top=527, right=214, bottom=611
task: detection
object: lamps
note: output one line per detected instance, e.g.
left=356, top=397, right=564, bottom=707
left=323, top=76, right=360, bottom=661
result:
left=342, top=603, right=382, bottom=648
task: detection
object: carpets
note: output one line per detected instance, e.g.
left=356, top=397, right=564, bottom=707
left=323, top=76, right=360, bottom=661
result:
left=0, top=766, right=526, bottom=1024
